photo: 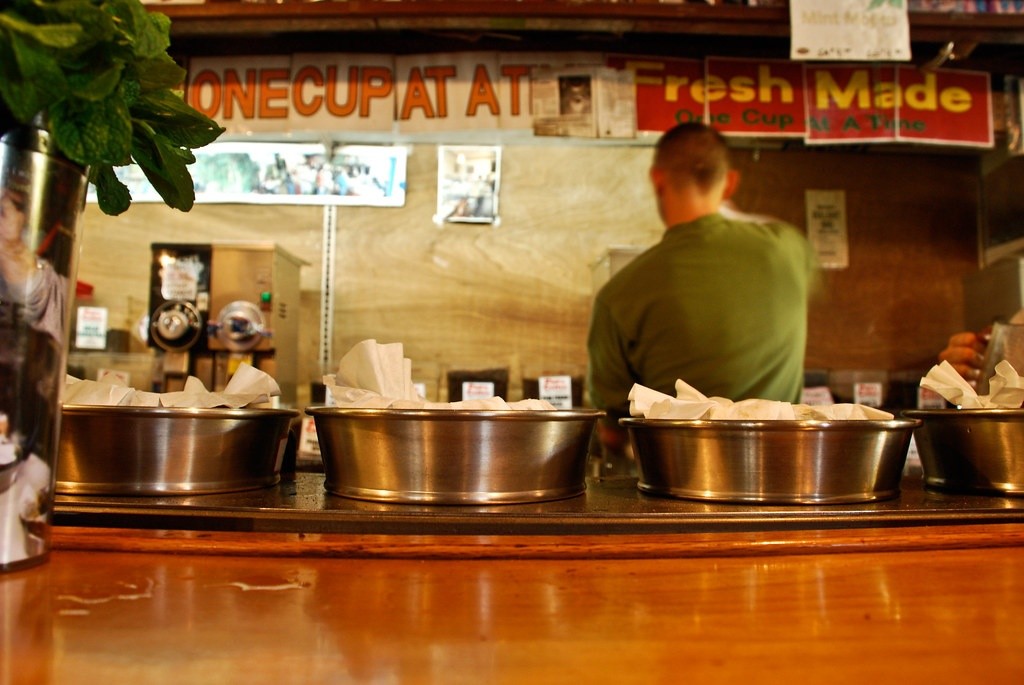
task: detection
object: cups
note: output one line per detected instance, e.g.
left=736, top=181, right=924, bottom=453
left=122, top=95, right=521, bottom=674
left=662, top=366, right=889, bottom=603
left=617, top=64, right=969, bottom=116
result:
left=975, top=322, right=1024, bottom=393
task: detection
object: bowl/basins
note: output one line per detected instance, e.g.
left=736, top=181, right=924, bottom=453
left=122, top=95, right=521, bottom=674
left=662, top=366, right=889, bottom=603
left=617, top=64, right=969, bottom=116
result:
left=303, top=407, right=607, bottom=505
left=899, top=408, right=1024, bottom=496
left=54, top=403, right=301, bottom=495
left=617, top=417, right=921, bottom=503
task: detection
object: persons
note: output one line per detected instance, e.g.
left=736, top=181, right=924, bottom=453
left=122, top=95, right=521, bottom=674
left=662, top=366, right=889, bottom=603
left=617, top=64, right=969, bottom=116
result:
left=587, top=121, right=816, bottom=460
left=937, top=308, right=1024, bottom=395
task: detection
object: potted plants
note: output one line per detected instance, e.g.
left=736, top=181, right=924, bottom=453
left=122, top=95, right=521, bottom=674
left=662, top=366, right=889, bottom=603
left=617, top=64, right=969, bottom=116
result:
left=0, top=0, right=229, bottom=572
